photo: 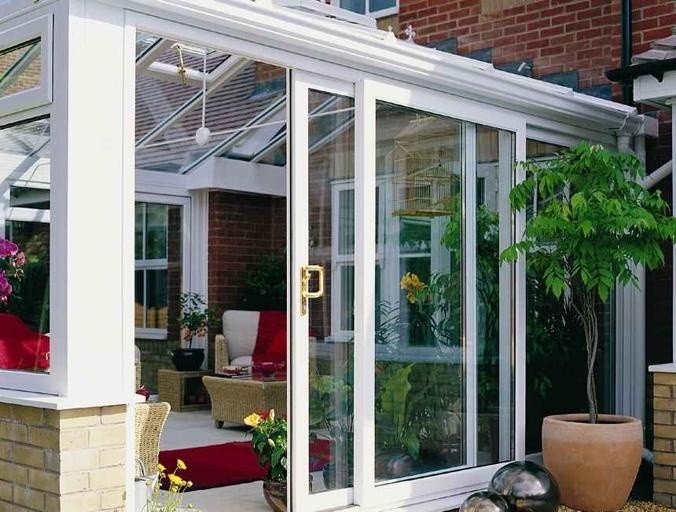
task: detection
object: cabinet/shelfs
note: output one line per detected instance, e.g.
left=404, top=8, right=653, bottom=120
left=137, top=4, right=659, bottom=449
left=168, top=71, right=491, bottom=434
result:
left=159, top=369, right=210, bottom=412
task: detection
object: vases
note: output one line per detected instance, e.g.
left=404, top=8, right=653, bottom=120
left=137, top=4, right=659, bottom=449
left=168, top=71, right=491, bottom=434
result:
left=262, top=474, right=313, bottom=511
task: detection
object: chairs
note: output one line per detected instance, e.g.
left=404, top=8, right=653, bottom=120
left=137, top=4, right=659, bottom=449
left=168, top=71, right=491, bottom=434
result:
left=215, top=311, right=286, bottom=381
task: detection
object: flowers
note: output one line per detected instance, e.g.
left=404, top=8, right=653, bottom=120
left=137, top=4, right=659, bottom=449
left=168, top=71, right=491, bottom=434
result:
left=242, top=408, right=287, bottom=480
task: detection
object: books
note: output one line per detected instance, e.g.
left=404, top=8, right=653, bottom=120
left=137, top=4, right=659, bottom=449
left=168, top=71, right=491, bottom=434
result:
left=214, top=373, right=249, bottom=379
left=221, top=364, right=250, bottom=375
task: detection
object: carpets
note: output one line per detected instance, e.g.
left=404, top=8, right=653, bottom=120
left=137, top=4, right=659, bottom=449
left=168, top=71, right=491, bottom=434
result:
left=158, top=438, right=334, bottom=493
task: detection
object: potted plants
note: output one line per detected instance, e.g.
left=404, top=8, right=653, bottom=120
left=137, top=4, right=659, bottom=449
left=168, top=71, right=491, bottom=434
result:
left=168, top=289, right=225, bottom=372
left=311, top=361, right=499, bottom=489
left=499, top=137, right=676, bottom=512
